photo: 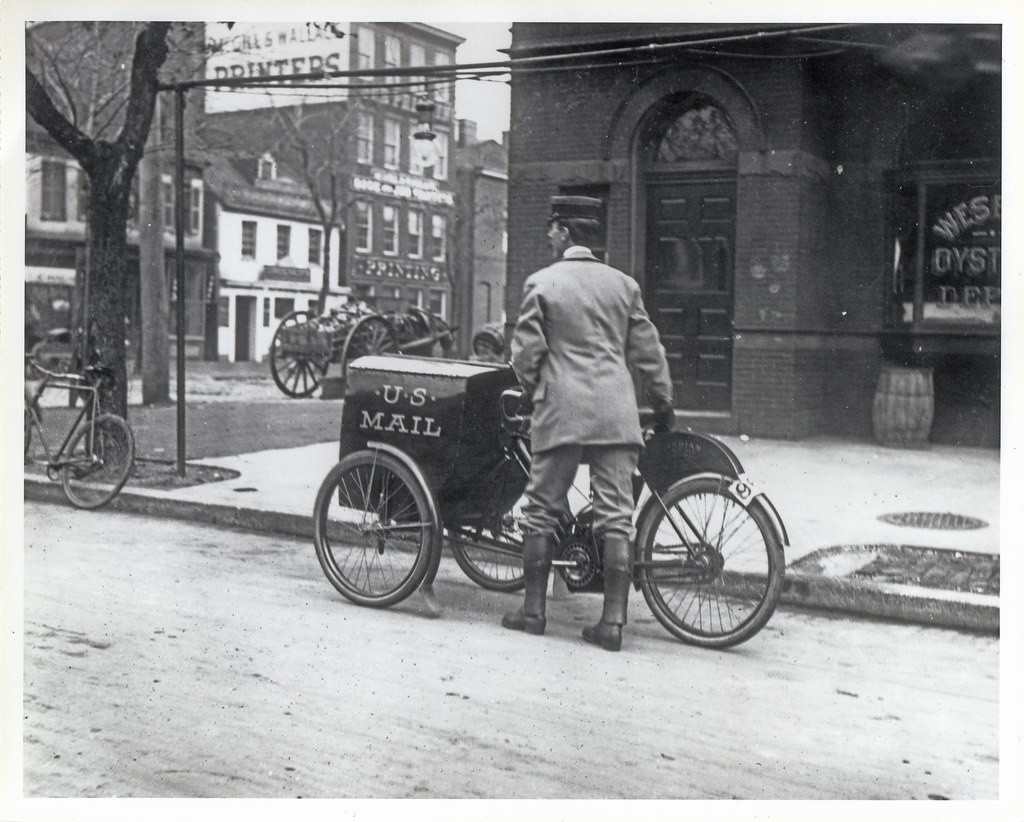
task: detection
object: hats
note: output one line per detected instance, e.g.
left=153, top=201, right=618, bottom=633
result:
left=544, top=195, right=604, bottom=223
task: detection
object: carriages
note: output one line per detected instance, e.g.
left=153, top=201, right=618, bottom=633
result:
left=267, top=295, right=461, bottom=399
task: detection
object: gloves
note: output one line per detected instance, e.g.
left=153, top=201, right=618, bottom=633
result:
left=653, top=409, right=676, bottom=435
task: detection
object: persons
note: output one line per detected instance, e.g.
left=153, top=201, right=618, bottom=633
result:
left=501, top=195, right=673, bottom=650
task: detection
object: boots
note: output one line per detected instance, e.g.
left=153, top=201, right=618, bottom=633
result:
left=581, top=538, right=632, bottom=651
left=501, top=535, right=554, bottom=635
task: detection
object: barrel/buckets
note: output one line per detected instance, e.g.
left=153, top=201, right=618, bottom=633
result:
left=873, top=367, right=934, bottom=448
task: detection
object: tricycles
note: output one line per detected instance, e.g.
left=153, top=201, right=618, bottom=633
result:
left=312, top=355, right=791, bottom=649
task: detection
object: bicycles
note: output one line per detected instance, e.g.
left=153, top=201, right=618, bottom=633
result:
left=24, top=326, right=136, bottom=512
